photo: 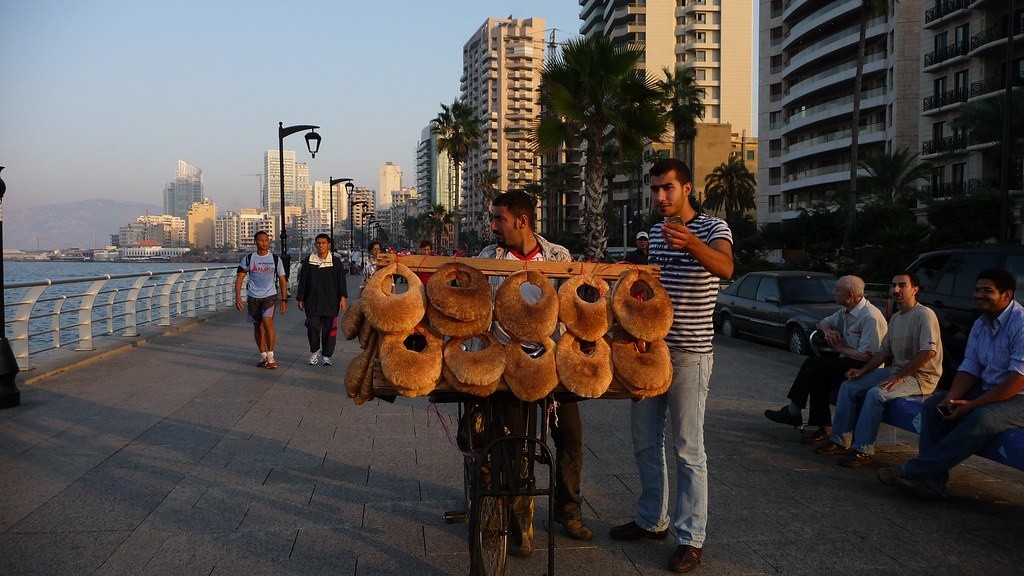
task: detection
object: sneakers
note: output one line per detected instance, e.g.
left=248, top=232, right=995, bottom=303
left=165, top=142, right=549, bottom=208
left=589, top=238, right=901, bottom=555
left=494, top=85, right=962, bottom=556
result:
left=802, top=428, right=831, bottom=444
left=256, top=359, right=265, bottom=366
left=506, top=531, right=532, bottom=557
left=839, top=450, right=872, bottom=466
left=610, top=520, right=668, bottom=540
left=265, top=358, right=277, bottom=369
left=764, top=405, right=803, bottom=426
left=308, top=348, right=321, bottom=365
left=670, top=544, right=702, bottom=572
left=321, top=356, right=332, bottom=366
left=557, top=520, right=593, bottom=540
left=815, top=441, right=852, bottom=454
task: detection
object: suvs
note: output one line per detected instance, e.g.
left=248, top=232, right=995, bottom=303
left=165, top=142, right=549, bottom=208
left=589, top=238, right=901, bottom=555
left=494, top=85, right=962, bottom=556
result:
left=885, top=248, right=1024, bottom=373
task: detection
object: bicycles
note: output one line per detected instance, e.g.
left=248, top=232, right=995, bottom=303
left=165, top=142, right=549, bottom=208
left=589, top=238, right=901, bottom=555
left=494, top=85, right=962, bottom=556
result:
left=429, top=389, right=563, bottom=576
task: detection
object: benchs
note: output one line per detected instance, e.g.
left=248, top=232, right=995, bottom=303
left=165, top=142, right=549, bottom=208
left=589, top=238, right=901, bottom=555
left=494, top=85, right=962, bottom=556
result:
left=808, top=322, right=1024, bottom=475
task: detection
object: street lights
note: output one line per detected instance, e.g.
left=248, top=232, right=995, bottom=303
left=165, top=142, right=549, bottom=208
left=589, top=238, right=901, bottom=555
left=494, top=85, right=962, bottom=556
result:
left=276, top=121, right=323, bottom=297
left=350, top=200, right=369, bottom=252
left=329, top=175, right=355, bottom=251
left=361, top=210, right=390, bottom=272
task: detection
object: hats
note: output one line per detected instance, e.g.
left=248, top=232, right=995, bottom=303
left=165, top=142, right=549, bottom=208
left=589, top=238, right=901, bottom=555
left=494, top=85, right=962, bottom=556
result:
left=636, top=231, right=649, bottom=240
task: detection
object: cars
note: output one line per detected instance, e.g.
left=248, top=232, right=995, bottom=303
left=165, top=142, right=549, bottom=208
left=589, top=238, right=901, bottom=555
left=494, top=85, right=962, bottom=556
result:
left=576, top=247, right=640, bottom=290
left=713, top=271, right=848, bottom=355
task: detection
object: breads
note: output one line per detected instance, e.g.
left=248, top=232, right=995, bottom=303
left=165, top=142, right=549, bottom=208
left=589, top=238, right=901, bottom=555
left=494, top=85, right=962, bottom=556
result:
left=341, top=262, right=673, bottom=404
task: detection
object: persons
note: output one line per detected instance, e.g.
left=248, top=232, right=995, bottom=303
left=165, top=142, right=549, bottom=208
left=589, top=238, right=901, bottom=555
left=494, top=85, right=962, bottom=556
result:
left=461, top=188, right=592, bottom=555
left=235, top=231, right=287, bottom=369
left=296, top=233, right=348, bottom=366
left=415, top=240, right=437, bottom=290
left=815, top=272, right=943, bottom=468
left=764, top=275, right=888, bottom=445
left=350, top=248, right=362, bottom=275
left=358, top=241, right=396, bottom=299
left=609, top=158, right=734, bottom=572
left=877, top=267, right=1024, bottom=495
left=625, top=231, right=650, bottom=265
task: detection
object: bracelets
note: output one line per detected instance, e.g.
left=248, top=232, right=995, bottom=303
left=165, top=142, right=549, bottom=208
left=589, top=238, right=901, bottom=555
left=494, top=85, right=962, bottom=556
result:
left=282, top=299, right=287, bottom=303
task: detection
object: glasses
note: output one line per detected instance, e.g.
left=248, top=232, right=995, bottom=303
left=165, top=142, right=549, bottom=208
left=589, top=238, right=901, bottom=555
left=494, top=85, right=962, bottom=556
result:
left=974, top=288, right=1001, bottom=294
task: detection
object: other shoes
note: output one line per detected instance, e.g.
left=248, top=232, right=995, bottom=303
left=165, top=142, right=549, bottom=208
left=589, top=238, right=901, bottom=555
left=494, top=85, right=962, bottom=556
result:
left=877, top=465, right=935, bottom=504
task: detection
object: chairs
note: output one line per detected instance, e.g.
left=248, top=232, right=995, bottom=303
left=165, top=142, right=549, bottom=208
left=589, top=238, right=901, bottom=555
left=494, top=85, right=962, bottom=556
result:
left=343, top=262, right=351, bottom=276
